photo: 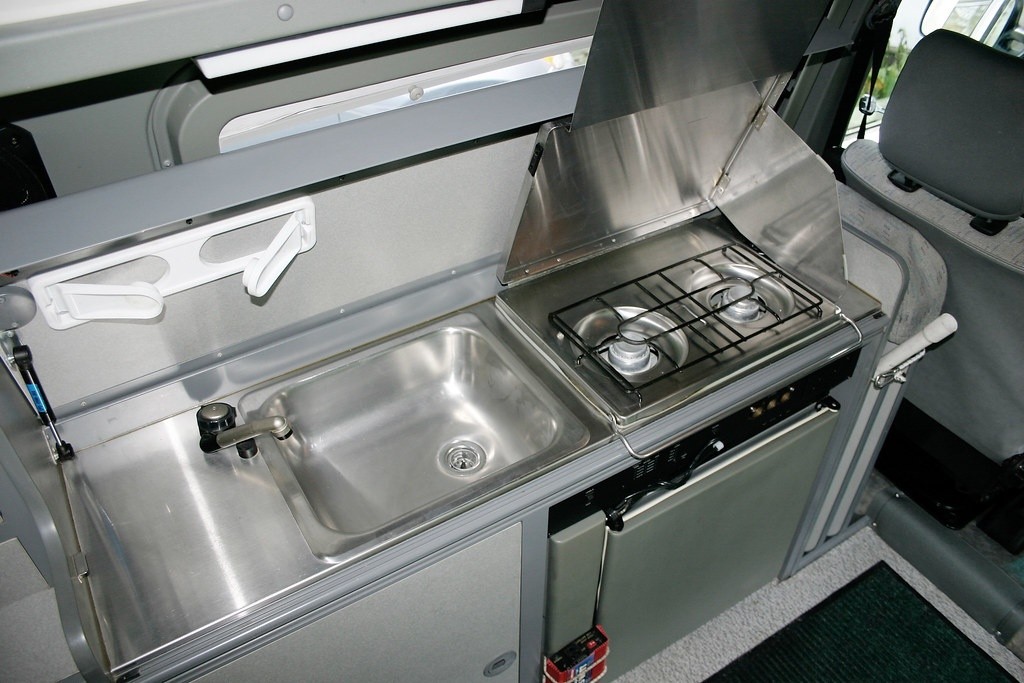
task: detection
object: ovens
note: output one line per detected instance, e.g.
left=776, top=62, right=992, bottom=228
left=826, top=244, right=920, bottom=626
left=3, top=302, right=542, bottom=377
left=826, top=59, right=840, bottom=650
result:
left=546, top=397, right=840, bottom=683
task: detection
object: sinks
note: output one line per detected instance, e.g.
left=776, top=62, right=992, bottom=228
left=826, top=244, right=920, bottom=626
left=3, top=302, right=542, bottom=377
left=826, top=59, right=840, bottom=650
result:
left=265, top=328, right=592, bottom=540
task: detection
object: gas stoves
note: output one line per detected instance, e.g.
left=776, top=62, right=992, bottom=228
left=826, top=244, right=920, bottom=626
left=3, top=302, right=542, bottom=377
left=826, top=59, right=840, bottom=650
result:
left=494, top=218, right=891, bottom=425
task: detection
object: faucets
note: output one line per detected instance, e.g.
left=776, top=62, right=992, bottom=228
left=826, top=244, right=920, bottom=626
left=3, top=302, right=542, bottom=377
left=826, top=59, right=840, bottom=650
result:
left=194, top=399, right=306, bottom=453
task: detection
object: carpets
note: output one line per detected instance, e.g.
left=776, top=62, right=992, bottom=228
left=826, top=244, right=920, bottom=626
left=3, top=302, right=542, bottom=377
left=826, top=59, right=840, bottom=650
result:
left=703, top=561, right=1022, bottom=682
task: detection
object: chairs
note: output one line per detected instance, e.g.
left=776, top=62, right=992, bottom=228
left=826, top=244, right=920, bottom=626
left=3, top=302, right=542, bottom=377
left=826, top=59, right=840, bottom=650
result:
left=843, top=25, right=1024, bottom=554
